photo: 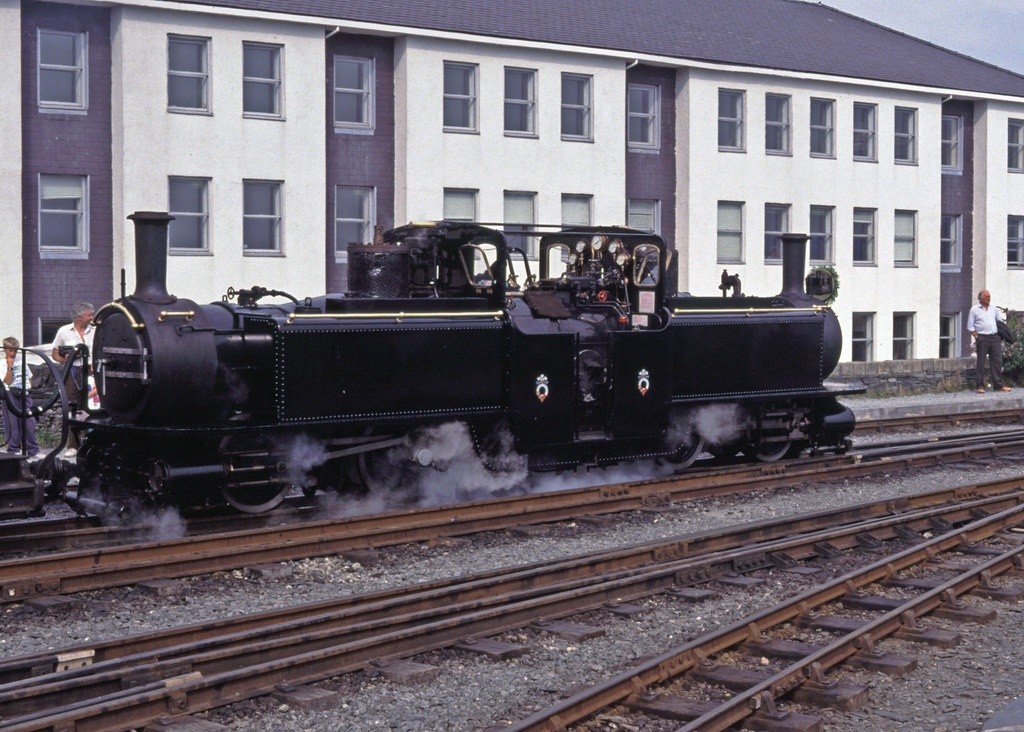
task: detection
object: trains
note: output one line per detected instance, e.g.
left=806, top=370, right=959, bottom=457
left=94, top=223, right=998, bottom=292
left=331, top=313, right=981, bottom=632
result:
left=0, top=205, right=875, bottom=519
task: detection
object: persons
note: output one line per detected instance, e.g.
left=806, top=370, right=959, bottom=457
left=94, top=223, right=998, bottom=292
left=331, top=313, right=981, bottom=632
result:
left=86, top=361, right=101, bottom=411
left=967, top=290, right=1013, bottom=393
left=51, top=302, right=97, bottom=458
left=0, top=336, right=48, bottom=460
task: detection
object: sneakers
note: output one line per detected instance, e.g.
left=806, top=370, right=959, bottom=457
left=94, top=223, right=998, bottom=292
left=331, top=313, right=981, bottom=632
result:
left=975, top=387, right=985, bottom=393
left=996, top=386, right=1011, bottom=391
left=64, top=448, right=79, bottom=459
left=31, top=452, right=47, bottom=460
